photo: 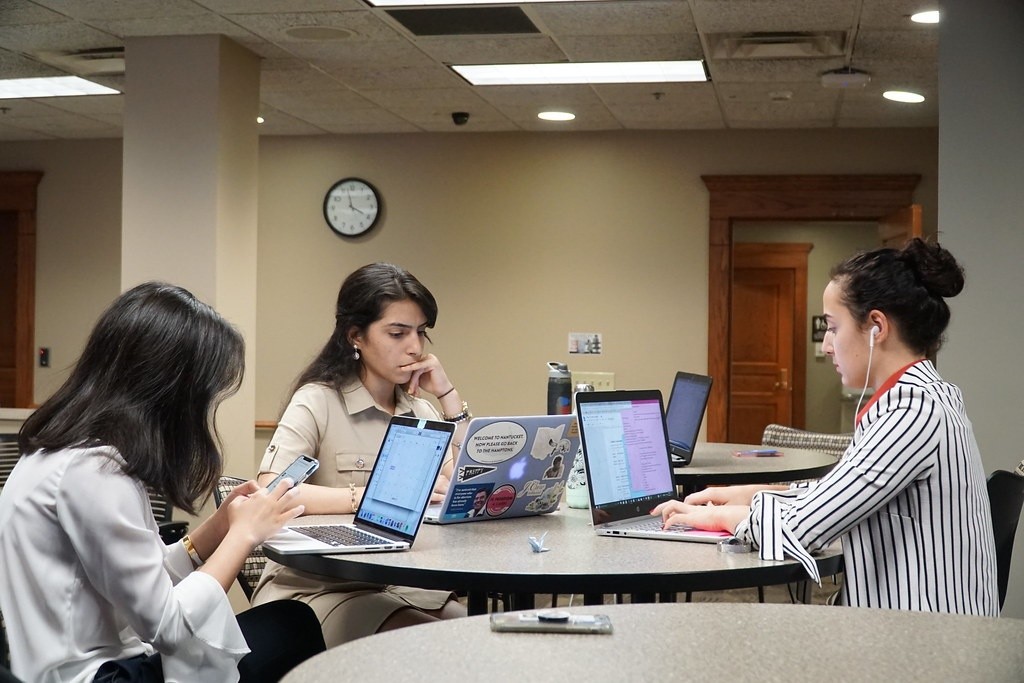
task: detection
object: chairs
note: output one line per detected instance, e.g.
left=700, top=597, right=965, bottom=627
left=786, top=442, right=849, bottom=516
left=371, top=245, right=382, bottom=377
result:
left=212, top=475, right=269, bottom=602
left=985, top=469, right=1024, bottom=613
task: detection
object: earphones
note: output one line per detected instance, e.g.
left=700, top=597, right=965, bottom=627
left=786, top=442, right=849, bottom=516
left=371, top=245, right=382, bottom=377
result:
left=869, top=325, right=880, bottom=347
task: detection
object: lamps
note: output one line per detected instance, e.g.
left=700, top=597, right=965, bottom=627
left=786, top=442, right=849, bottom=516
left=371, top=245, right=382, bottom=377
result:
left=819, top=66, right=871, bottom=89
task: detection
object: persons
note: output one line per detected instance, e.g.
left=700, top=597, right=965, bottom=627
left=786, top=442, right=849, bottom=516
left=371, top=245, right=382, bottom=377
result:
left=649, top=237, right=1000, bottom=617
left=250, top=263, right=471, bottom=648
left=0, top=282, right=326, bottom=683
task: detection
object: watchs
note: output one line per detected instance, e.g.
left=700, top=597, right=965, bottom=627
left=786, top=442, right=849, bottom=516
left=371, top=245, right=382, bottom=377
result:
left=441, top=400, right=472, bottom=423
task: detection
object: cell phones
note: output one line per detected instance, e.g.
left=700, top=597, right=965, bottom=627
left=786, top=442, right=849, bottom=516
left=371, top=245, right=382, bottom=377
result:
left=490, top=615, right=612, bottom=636
left=266, top=454, right=319, bottom=492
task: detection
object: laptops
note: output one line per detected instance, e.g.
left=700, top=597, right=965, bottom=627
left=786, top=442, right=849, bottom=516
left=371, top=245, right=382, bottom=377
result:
left=574, top=390, right=736, bottom=543
left=665, top=372, right=713, bottom=467
left=261, top=416, right=457, bottom=555
left=423, top=414, right=581, bottom=525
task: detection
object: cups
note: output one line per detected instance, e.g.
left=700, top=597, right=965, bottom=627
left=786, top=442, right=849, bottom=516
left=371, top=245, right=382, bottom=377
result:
left=566, top=445, right=590, bottom=508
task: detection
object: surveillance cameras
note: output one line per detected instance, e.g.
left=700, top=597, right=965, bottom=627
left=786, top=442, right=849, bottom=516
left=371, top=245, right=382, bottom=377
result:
left=452, top=112, right=470, bottom=125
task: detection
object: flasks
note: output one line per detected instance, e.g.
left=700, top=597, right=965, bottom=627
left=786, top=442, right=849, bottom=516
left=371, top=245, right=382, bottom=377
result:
left=572, top=385, right=594, bottom=416
left=546, top=362, right=572, bottom=416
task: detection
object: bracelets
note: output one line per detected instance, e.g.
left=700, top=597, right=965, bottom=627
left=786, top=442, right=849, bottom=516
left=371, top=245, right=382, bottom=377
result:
left=350, top=483, right=358, bottom=513
left=183, top=535, right=204, bottom=566
left=437, top=387, right=455, bottom=399
left=452, top=442, right=461, bottom=448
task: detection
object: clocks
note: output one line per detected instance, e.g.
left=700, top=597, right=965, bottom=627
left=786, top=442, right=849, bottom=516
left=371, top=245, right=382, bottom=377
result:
left=323, top=177, right=382, bottom=239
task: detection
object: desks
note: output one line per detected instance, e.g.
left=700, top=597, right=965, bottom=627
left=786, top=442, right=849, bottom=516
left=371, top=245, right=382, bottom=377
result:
left=256, top=493, right=846, bottom=618
left=669, top=442, right=841, bottom=503
left=275, top=601, right=1024, bottom=683
